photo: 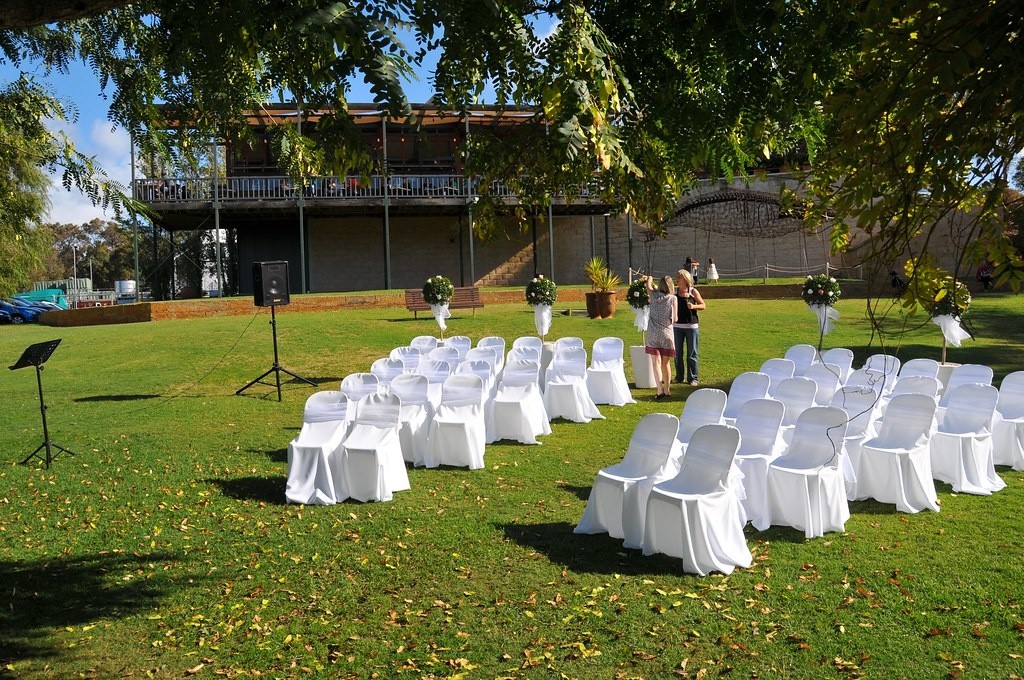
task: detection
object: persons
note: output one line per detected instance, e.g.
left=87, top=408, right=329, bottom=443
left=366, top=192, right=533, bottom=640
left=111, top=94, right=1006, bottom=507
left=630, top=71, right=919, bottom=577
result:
left=446, top=178, right=460, bottom=193
left=323, top=174, right=336, bottom=192
left=707, top=257, right=720, bottom=286
left=643, top=269, right=705, bottom=387
left=149, top=173, right=174, bottom=200
left=424, top=179, right=436, bottom=192
left=644, top=275, right=678, bottom=399
left=387, top=176, right=394, bottom=188
left=684, top=256, right=699, bottom=284
left=402, top=176, right=412, bottom=192
left=280, top=178, right=290, bottom=198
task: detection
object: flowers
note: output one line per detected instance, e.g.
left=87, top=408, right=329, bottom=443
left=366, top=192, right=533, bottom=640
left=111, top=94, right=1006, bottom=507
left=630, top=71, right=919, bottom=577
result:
left=420, top=275, right=456, bottom=307
left=800, top=274, right=842, bottom=306
left=928, top=279, right=971, bottom=317
left=626, top=277, right=658, bottom=308
left=523, top=274, right=559, bottom=306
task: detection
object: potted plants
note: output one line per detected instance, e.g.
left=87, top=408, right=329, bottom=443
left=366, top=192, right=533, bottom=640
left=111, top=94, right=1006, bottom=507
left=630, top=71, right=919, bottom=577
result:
left=593, top=268, right=623, bottom=319
left=584, top=255, right=606, bottom=319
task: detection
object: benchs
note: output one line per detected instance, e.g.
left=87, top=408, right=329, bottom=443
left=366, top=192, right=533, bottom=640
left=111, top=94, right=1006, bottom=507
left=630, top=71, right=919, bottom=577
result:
left=405, top=286, right=485, bottom=319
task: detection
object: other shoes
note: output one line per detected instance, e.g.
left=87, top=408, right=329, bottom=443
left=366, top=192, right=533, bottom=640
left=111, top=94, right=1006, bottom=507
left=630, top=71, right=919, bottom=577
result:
left=654, top=394, right=664, bottom=398
left=690, top=380, right=699, bottom=386
left=670, top=378, right=679, bottom=383
left=663, top=393, right=670, bottom=398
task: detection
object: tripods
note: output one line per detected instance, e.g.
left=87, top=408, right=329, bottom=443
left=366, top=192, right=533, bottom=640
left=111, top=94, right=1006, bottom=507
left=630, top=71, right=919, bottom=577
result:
left=7, top=339, right=75, bottom=470
left=236, top=305, right=319, bottom=403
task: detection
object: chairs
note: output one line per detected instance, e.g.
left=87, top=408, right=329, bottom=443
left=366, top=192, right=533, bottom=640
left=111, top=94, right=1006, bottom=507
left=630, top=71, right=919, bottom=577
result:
left=859, top=393, right=936, bottom=514
left=831, top=385, right=877, bottom=500
left=587, top=336, right=636, bottom=406
left=861, top=354, right=901, bottom=391
left=898, top=358, right=939, bottom=380
left=992, top=371, right=1024, bottom=471
left=773, top=377, right=819, bottom=444
left=783, top=344, right=816, bottom=377
left=676, top=388, right=726, bottom=467
left=286, top=334, right=543, bottom=507
left=759, top=358, right=795, bottom=401
left=648, top=424, right=742, bottom=574
left=545, top=347, right=587, bottom=422
left=553, top=336, right=584, bottom=354
left=803, top=363, right=841, bottom=406
left=820, top=348, right=853, bottom=386
left=227, top=175, right=505, bottom=195
left=877, top=377, right=937, bottom=435
left=930, top=382, right=999, bottom=492
left=592, top=412, right=680, bottom=539
left=735, top=398, right=785, bottom=527
left=940, top=364, right=993, bottom=406
left=723, top=372, right=770, bottom=425
left=846, top=369, right=886, bottom=425
left=766, top=406, right=850, bottom=534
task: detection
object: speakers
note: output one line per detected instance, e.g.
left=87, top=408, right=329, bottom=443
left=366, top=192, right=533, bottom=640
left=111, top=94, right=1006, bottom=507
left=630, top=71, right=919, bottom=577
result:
left=253, top=261, right=291, bottom=307
